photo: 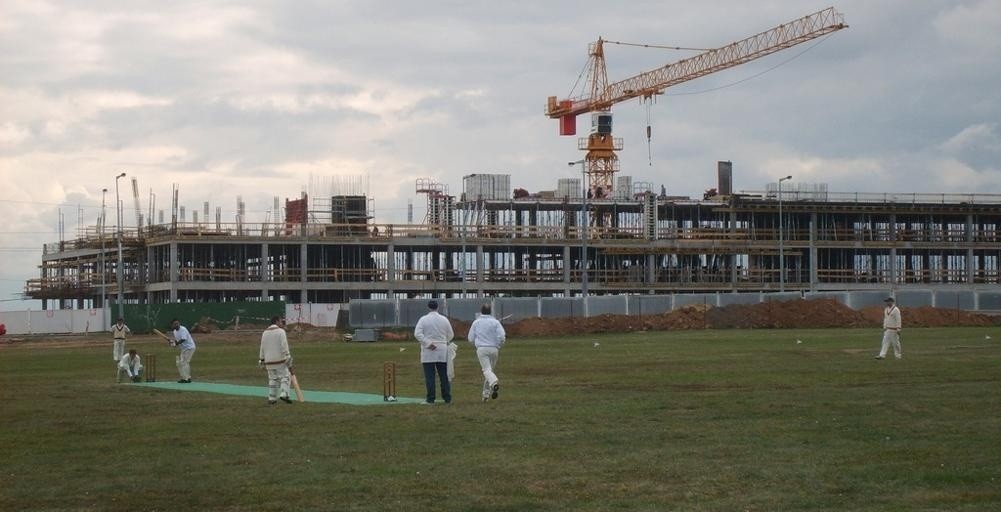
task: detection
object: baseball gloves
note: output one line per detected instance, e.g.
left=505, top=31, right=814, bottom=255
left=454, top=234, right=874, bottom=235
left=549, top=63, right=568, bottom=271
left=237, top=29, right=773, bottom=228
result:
left=131, top=375, right=141, bottom=382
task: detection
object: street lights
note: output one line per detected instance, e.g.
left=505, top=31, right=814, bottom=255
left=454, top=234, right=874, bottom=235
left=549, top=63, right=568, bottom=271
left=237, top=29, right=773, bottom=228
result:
left=460, top=173, right=478, bottom=296
left=568, top=157, right=589, bottom=315
left=777, top=173, right=793, bottom=293
left=114, top=169, right=130, bottom=321
left=98, top=189, right=109, bottom=319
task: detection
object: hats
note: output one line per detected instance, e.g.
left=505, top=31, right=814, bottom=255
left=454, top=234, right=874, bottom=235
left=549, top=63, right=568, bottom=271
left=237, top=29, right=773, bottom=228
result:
left=884, top=298, right=894, bottom=302
left=128, top=350, right=136, bottom=354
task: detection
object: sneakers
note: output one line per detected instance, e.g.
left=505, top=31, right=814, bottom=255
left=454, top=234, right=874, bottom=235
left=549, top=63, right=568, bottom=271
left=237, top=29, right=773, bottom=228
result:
left=491, top=385, right=499, bottom=399
left=875, top=355, right=885, bottom=359
left=420, top=400, right=435, bottom=405
left=177, top=379, right=188, bottom=383
left=279, top=397, right=291, bottom=404
left=188, top=378, right=190, bottom=383
left=268, top=400, right=276, bottom=404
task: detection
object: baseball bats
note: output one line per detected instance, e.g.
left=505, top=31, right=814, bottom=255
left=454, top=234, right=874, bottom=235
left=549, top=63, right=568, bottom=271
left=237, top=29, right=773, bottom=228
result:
left=288, top=368, right=304, bottom=404
left=153, top=329, right=174, bottom=344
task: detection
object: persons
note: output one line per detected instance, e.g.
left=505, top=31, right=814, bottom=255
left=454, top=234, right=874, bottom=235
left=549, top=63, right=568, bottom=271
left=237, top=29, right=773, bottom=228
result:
left=876, top=298, right=903, bottom=361
left=258, top=314, right=294, bottom=404
left=110, top=317, right=130, bottom=361
left=412, top=298, right=455, bottom=405
left=114, top=350, right=145, bottom=383
left=167, top=319, right=197, bottom=382
left=467, top=303, right=506, bottom=402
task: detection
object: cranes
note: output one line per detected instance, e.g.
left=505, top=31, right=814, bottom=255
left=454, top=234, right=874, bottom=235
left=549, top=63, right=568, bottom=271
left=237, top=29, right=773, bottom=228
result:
left=543, top=7, right=849, bottom=239
